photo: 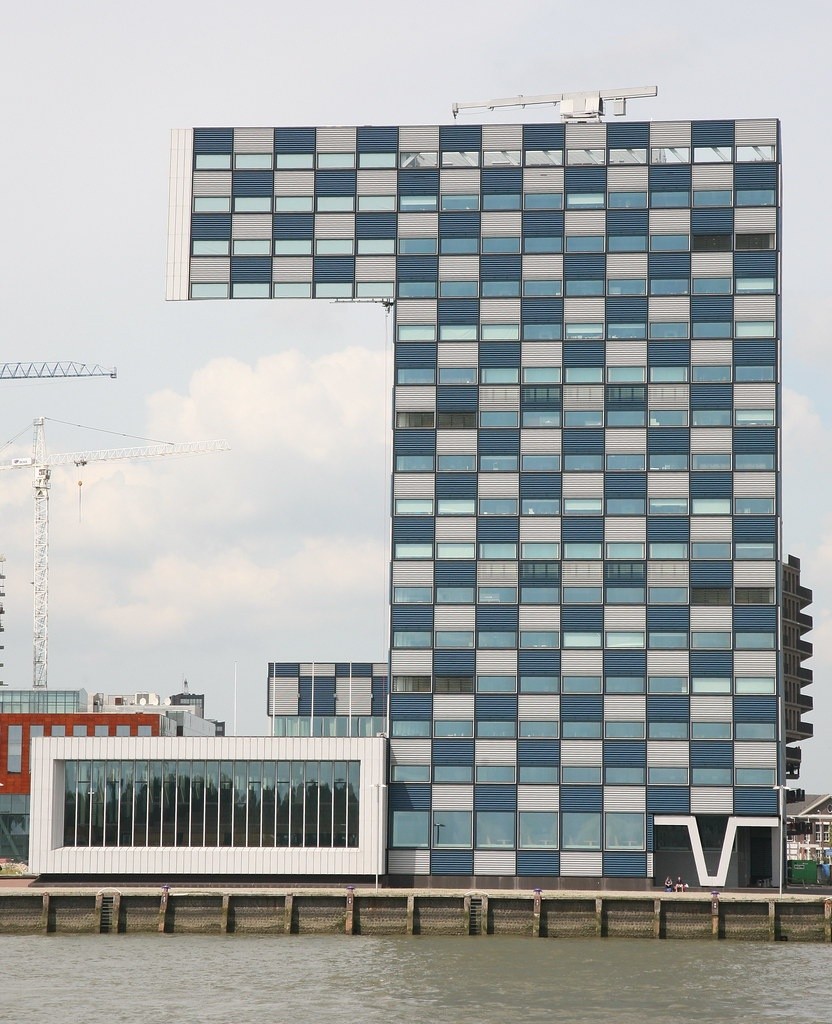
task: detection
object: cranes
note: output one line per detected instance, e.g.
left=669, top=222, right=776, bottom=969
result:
left=0, top=359, right=119, bottom=688
left=0, top=416, right=233, bottom=691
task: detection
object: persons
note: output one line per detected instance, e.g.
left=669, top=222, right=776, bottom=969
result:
left=665, top=876, right=684, bottom=892
